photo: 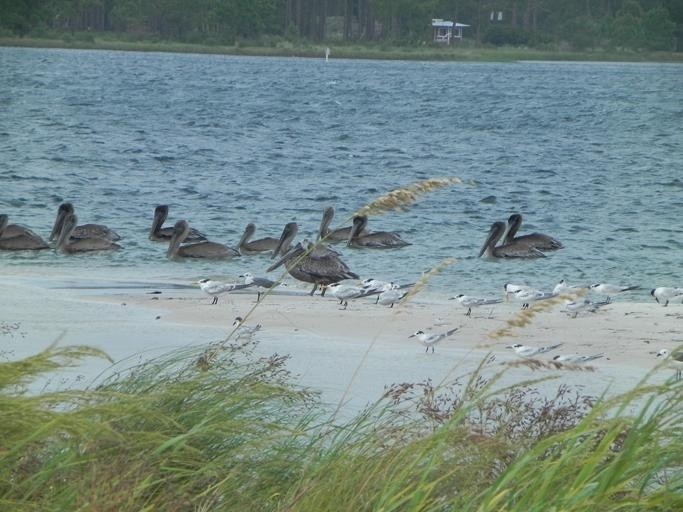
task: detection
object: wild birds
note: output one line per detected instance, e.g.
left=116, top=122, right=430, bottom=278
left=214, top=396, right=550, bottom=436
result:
left=0, top=200, right=123, bottom=255
left=408, top=279, right=683, bottom=372
left=147, top=202, right=409, bottom=311
left=475, top=213, right=563, bottom=262
